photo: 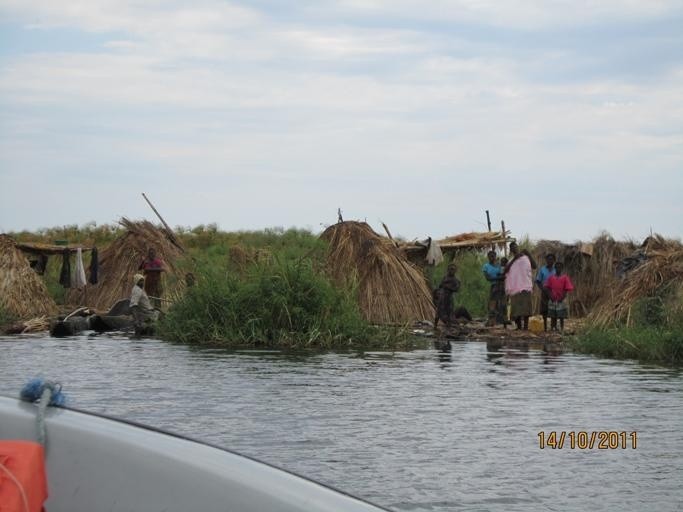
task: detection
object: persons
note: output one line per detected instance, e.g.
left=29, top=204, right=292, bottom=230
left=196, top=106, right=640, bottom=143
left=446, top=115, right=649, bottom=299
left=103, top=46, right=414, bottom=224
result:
left=505, top=243, right=537, bottom=331
left=493, top=258, right=508, bottom=291
left=543, top=263, right=573, bottom=332
left=534, top=254, right=558, bottom=332
left=138, top=248, right=168, bottom=308
left=433, top=264, right=460, bottom=327
left=184, top=273, right=197, bottom=286
left=129, top=275, right=162, bottom=328
left=483, top=250, right=511, bottom=326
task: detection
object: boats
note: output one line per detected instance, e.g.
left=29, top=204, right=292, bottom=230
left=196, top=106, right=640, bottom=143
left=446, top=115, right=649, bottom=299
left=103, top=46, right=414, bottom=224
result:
left=0, top=395, right=395, bottom=512
left=52, top=297, right=167, bottom=337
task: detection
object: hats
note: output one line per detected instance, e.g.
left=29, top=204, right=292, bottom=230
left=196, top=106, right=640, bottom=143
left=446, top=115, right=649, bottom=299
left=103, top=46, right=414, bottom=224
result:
left=133, top=273, right=144, bottom=285
